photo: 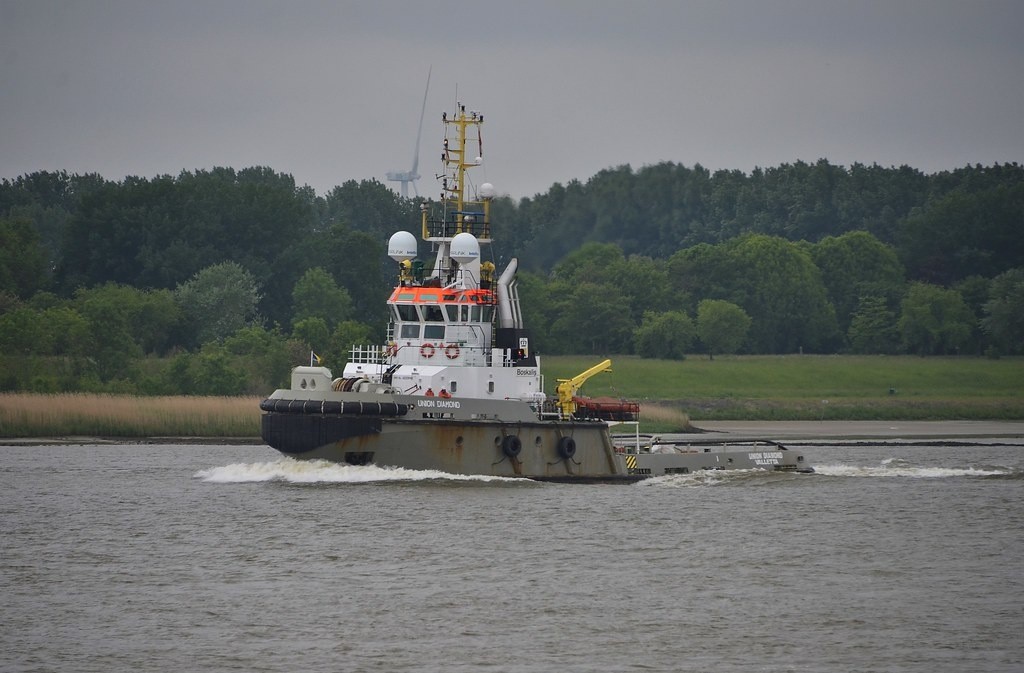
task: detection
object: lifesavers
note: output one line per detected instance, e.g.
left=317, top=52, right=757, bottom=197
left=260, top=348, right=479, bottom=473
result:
left=504, top=434, right=521, bottom=458
left=387, top=342, right=396, bottom=356
left=420, top=343, right=434, bottom=358
left=560, top=436, right=575, bottom=458
left=445, top=345, right=458, bottom=359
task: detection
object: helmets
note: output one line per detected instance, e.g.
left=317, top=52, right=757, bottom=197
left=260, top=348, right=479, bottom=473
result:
left=441, top=386, right=446, bottom=390
left=427, top=386, right=432, bottom=389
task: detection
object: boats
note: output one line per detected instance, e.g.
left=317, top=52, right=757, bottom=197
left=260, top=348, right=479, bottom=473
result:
left=258, top=101, right=815, bottom=484
left=572, top=394, right=641, bottom=413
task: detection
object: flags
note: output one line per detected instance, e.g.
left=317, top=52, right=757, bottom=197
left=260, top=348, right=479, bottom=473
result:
left=313, top=354, right=321, bottom=364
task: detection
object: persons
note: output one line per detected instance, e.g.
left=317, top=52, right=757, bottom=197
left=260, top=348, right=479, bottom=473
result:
left=425, top=386, right=434, bottom=397
left=439, top=386, right=451, bottom=398
